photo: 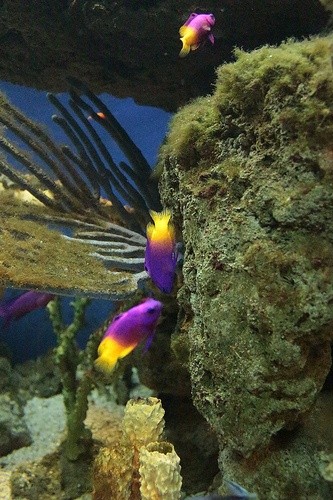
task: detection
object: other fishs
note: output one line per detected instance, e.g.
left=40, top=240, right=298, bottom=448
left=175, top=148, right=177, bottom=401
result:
left=93, top=296, right=165, bottom=377
left=179, top=12, right=216, bottom=58
left=87, top=112, right=106, bottom=120
left=0, top=289, right=54, bottom=330
left=183, top=480, right=258, bottom=500
left=144, top=209, right=184, bottom=294
